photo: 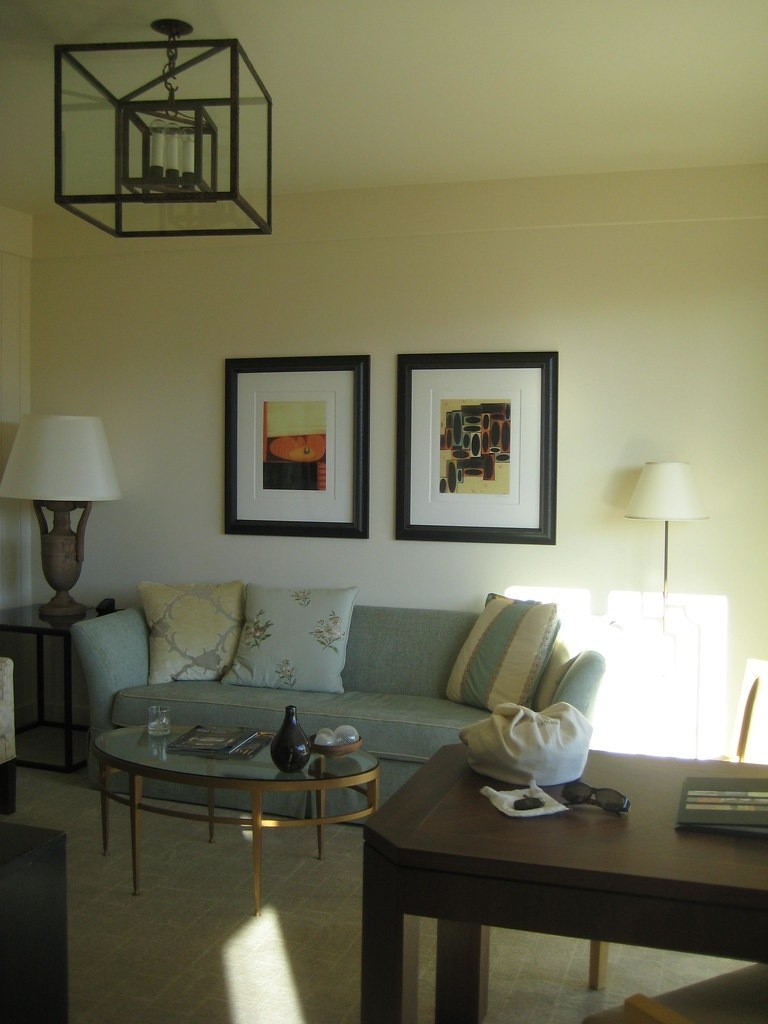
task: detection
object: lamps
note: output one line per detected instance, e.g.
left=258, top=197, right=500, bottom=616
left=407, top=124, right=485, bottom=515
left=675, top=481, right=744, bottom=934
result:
left=624, top=461, right=709, bottom=635
left=0, top=416, right=123, bottom=617
left=54, top=18, right=274, bottom=238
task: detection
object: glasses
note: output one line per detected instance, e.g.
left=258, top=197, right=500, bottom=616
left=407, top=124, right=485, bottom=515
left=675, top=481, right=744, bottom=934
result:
left=562, top=780, right=631, bottom=814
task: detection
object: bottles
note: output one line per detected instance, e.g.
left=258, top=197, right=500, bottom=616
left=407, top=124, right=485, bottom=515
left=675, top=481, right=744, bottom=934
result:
left=270, top=705, right=310, bottom=772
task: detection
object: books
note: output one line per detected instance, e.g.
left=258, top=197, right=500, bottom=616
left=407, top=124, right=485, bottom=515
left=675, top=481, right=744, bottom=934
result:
left=673, top=776, right=768, bottom=837
left=164, top=724, right=278, bottom=761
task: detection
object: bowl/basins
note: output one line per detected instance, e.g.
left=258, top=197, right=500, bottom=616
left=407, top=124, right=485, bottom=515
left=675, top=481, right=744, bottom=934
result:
left=308, top=734, right=363, bottom=759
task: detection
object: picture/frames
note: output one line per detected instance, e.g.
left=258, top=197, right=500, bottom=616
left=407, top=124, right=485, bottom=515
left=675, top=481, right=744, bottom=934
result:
left=224, top=355, right=371, bottom=539
left=396, top=352, right=559, bottom=545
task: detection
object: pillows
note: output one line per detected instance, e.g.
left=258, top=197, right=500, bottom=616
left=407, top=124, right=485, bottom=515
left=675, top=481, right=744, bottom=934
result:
left=220, top=583, right=371, bottom=695
left=136, top=580, right=245, bottom=685
left=446, top=593, right=560, bottom=712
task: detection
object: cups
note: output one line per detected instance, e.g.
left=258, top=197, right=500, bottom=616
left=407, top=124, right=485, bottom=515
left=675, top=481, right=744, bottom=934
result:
left=148, top=706, right=172, bottom=736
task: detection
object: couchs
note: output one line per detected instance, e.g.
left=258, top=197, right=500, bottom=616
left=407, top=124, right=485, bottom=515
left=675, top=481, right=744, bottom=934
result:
left=70, top=605, right=599, bottom=824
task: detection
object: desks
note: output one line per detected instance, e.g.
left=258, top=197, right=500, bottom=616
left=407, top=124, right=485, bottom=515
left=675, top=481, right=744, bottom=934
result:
left=0, top=822, right=69, bottom=1024
left=0, top=603, right=126, bottom=773
left=361, top=744, right=768, bottom=1024
left=91, top=724, right=380, bottom=916
